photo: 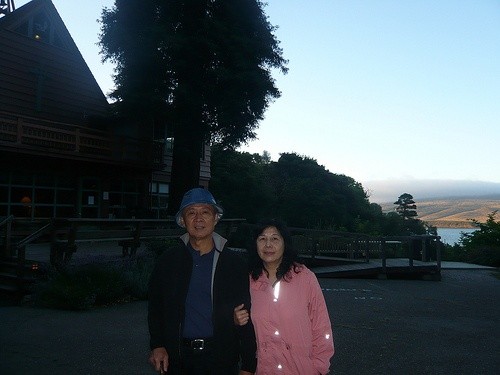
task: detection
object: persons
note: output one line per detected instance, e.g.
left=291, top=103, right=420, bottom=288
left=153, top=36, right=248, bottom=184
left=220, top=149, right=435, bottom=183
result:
left=145, top=187, right=258, bottom=375
left=232, top=217, right=335, bottom=375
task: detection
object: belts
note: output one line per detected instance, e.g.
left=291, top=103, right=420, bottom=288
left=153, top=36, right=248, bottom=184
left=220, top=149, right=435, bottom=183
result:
left=182, top=337, right=217, bottom=351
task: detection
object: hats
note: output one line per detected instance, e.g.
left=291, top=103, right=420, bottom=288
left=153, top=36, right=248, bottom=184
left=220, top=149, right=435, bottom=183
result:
left=175, top=188, right=223, bottom=228
